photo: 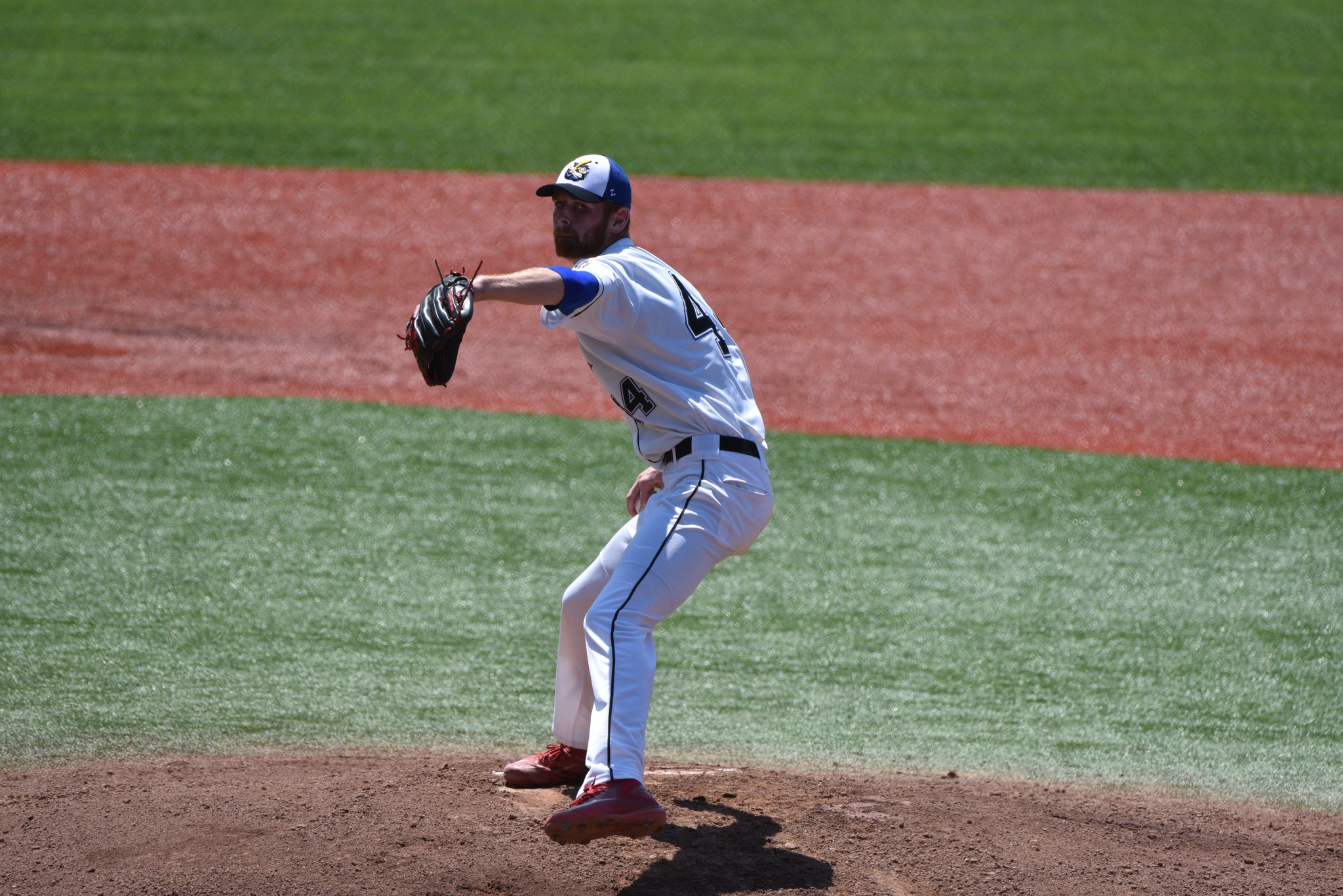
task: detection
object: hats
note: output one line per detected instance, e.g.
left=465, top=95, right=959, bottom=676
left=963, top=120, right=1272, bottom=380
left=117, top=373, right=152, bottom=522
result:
left=536, top=155, right=632, bottom=210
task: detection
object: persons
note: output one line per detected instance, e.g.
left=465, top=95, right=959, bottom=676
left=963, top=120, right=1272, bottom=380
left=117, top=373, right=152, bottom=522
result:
left=398, top=153, right=773, bottom=845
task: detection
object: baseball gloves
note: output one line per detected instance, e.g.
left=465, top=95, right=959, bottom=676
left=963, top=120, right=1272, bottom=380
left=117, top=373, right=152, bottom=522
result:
left=405, top=272, right=471, bottom=386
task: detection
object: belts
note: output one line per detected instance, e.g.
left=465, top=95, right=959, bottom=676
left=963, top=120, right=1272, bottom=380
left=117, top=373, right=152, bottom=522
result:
left=662, top=434, right=760, bottom=467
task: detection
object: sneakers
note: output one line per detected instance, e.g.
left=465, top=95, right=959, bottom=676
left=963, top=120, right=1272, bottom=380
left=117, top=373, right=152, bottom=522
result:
left=504, top=742, right=590, bottom=786
left=544, top=778, right=667, bottom=846
